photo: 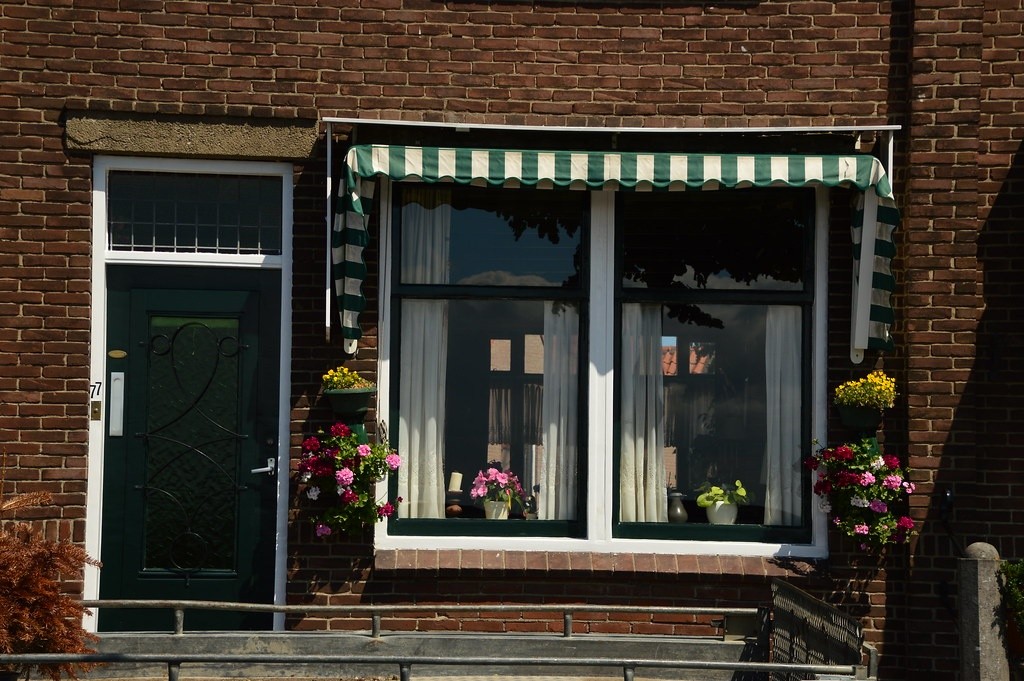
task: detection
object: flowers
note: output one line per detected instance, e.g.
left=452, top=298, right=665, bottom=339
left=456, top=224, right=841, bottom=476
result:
left=697, top=480, right=752, bottom=507
left=321, top=366, right=374, bottom=389
left=836, top=371, right=899, bottom=411
left=288, top=426, right=394, bottom=537
left=470, top=467, right=525, bottom=500
left=804, top=434, right=917, bottom=549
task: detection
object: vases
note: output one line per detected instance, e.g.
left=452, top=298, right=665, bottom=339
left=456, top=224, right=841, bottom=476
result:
left=837, top=405, right=884, bottom=429
left=325, top=391, right=376, bottom=418
left=837, top=489, right=872, bottom=516
left=708, top=503, right=738, bottom=524
left=668, top=493, right=688, bottom=523
left=316, top=479, right=346, bottom=497
left=483, top=501, right=511, bottom=520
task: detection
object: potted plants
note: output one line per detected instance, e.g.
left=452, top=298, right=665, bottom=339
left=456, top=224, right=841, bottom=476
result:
left=526, top=497, right=537, bottom=520
